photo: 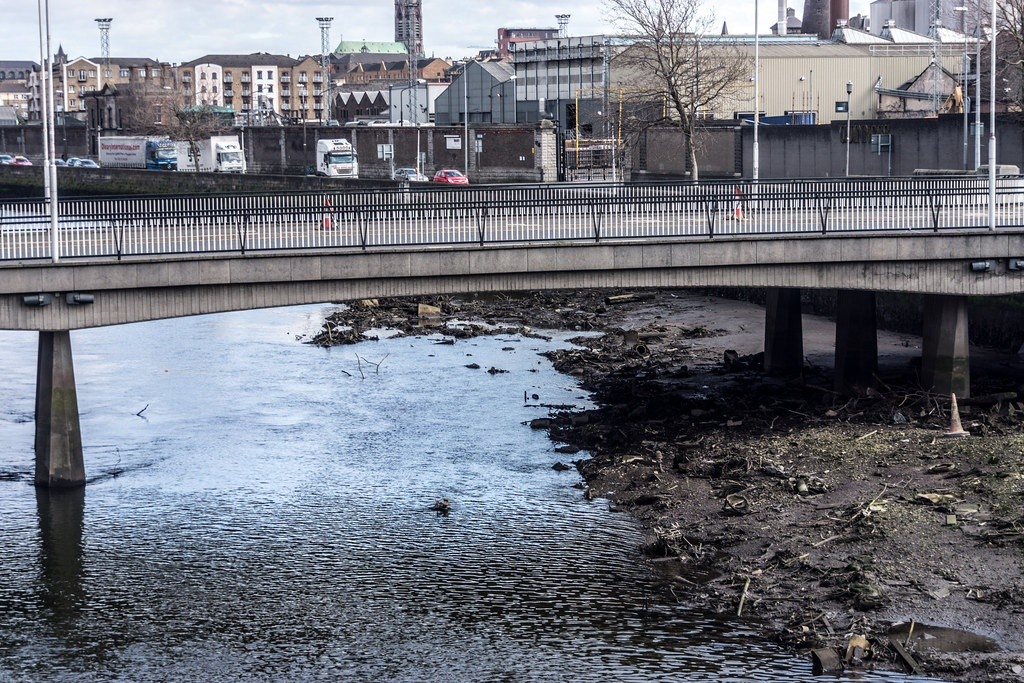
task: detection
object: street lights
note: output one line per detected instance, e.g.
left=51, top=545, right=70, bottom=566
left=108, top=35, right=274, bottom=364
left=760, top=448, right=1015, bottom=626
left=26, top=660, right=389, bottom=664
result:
left=490, top=76, right=517, bottom=124
left=95, top=18, right=114, bottom=85
left=247, top=86, right=271, bottom=126
left=456, top=61, right=467, bottom=176
left=846, top=81, right=853, bottom=175
left=555, top=15, right=572, bottom=39
left=319, top=82, right=344, bottom=126
left=317, top=17, right=335, bottom=122
left=401, top=80, right=426, bottom=126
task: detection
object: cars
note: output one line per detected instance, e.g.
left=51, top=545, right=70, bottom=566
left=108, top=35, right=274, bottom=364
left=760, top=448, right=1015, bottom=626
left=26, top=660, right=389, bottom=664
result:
left=0, top=155, right=12, bottom=164
left=394, top=168, right=429, bottom=181
left=433, top=170, right=468, bottom=185
left=74, top=159, right=98, bottom=168
left=52, top=159, right=65, bottom=165
left=329, top=120, right=339, bottom=126
left=357, top=120, right=390, bottom=126
left=9, top=156, right=32, bottom=165
left=68, top=158, right=79, bottom=166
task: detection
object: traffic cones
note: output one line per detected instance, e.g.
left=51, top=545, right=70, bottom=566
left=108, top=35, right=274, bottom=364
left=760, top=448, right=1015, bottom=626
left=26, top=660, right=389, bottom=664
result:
left=945, top=391, right=970, bottom=437
left=731, top=186, right=749, bottom=222
left=316, top=199, right=333, bottom=231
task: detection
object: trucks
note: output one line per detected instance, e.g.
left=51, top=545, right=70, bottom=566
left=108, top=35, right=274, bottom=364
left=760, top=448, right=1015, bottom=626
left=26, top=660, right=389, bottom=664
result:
left=316, top=139, right=358, bottom=178
left=175, top=135, right=247, bottom=174
left=99, top=135, right=177, bottom=170
